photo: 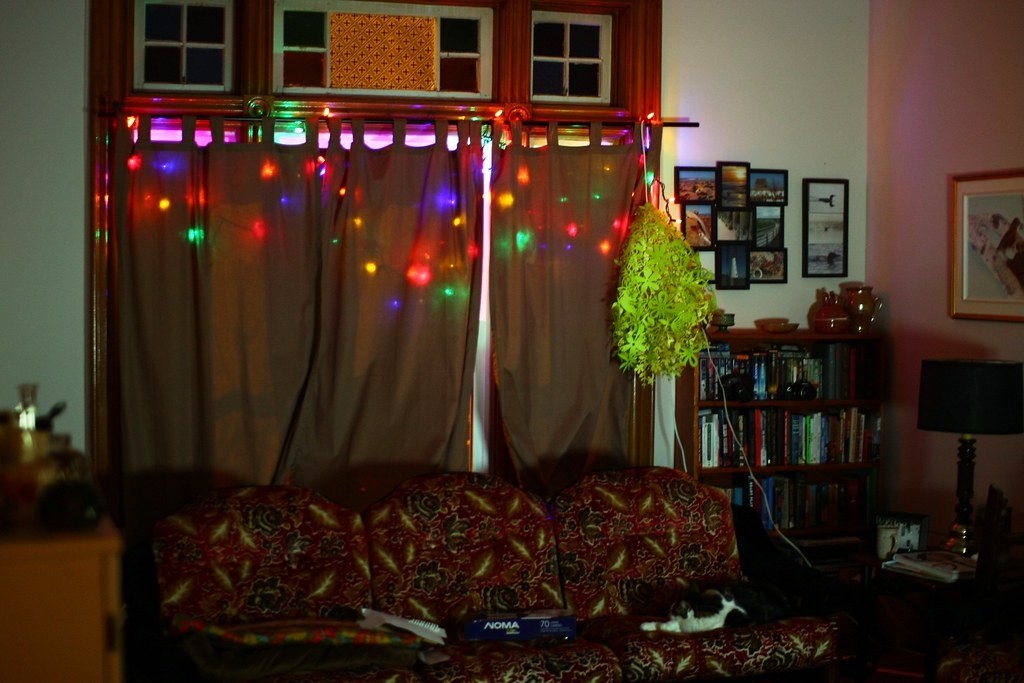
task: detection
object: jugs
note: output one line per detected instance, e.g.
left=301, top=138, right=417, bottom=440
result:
left=20, top=448, right=103, bottom=532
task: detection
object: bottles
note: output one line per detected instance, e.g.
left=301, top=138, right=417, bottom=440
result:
left=813, top=291, right=850, bottom=335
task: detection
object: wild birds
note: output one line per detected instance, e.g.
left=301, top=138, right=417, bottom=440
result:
left=996, top=218, right=1021, bottom=252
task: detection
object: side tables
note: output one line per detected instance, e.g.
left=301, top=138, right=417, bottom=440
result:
left=0, top=510, right=126, bottom=682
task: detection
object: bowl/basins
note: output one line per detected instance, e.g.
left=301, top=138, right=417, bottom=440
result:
left=760, top=323, right=800, bottom=333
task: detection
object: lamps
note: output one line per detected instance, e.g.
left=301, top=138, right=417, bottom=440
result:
left=917, top=358, right=1024, bottom=556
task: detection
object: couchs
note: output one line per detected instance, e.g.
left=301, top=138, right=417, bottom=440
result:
left=106, top=448, right=876, bottom=683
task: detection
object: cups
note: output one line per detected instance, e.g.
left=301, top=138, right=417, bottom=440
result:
left=876, top=524, right=900, bottom=560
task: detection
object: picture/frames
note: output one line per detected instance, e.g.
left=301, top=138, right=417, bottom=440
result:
left=801, top=177, right=849, bottom=277
left=674, top=161, right=789, bottom=291
left=947, top=166, right=1024, bottom=323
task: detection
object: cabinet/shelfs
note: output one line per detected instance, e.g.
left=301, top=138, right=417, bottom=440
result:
left=673, top=324, right=888, bottom=566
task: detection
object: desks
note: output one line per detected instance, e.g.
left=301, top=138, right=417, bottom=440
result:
left=852, top=548, right=1023, bottom=682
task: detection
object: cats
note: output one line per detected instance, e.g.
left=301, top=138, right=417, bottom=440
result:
left=640, top=584, right=748, bottom=632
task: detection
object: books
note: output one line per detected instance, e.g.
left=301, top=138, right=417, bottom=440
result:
left=698, top=408, right=880, bottom=467
left=894, top=552, right=975, bottom=579
left=701, top=343, right=857, bottom=400
left=704, top=475, right=859, bottom=531
